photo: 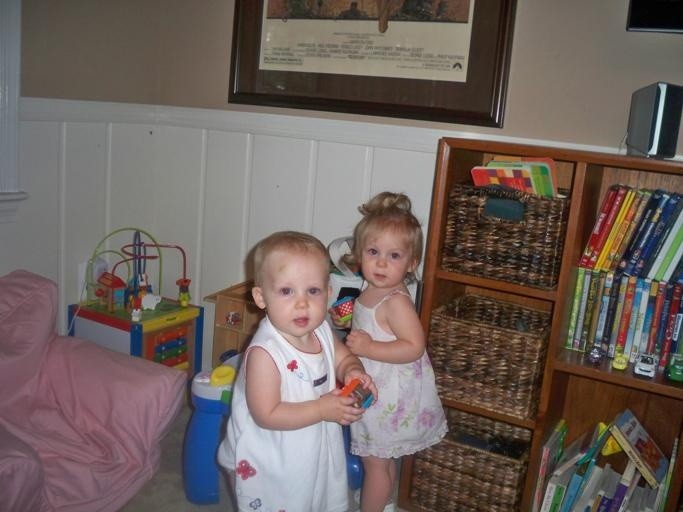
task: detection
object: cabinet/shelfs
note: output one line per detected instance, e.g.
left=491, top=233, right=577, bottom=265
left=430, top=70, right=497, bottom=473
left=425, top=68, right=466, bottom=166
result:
left=202, top=281, right=269, bottom=370
left=397, top=135, right=683, bottom=512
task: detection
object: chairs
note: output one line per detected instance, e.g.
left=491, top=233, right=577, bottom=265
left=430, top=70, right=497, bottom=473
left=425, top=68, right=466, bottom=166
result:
left=0, top=269, right=189, bottom=511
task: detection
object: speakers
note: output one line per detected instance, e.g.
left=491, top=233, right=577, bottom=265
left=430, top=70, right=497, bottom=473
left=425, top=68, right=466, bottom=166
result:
left=626, top=83, right=682, bottom=161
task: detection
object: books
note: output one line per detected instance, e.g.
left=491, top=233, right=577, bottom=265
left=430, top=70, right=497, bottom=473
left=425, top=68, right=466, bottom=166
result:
left=529, top=405, right=678, bottom=512
left=563, top=181, right=683, bottom=367
left=469, top=155, right=558, bottom=198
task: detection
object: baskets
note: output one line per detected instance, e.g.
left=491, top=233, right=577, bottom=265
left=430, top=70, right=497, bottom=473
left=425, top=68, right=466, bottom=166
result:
left=426, top=292, right=554, bottom=421
left=439, top=177, right=573, bottom=292
left=408, top=408, right=534, bottom=512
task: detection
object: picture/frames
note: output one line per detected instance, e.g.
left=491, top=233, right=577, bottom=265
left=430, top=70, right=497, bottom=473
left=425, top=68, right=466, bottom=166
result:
left=225, top=0, right=518, bottom=130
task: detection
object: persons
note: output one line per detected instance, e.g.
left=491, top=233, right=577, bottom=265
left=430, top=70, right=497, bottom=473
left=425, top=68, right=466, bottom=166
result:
left=212, top=231, right=379, bottom=511
left=327, top=191, right=451, bottom=510
